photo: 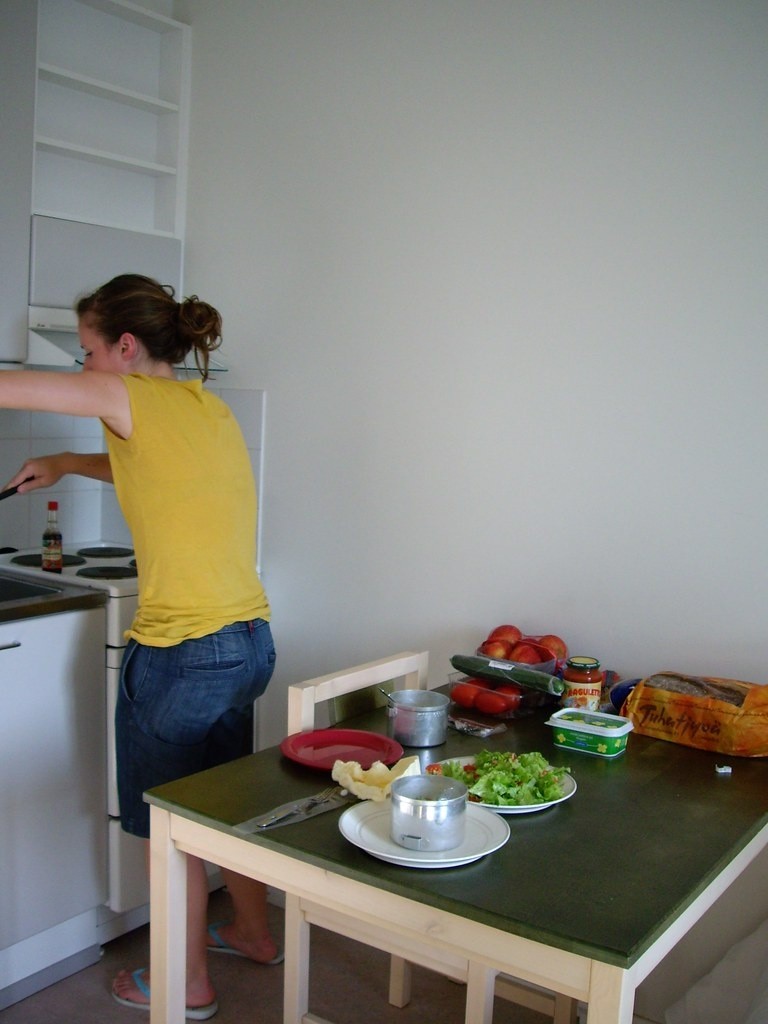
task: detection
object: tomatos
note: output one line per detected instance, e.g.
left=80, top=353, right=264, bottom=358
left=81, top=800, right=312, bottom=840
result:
left=481, top=625, right=566, bottom=664
left=451, top=679, right=521, bottom=712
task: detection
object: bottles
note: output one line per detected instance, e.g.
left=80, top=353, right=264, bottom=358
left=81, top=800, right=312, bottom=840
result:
left=559, top=656, right=602, bottom=711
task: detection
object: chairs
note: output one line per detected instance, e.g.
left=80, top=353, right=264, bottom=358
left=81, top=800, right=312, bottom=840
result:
left=286, top=648, right=434, bottom=1010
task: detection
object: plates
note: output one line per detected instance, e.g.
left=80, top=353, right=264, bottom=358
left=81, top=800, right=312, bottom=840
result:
left=437, top=757, right=578, bottom=814
left=281, top=729, right=405, bottom=770
left=338, top=796, right=511, bottom=868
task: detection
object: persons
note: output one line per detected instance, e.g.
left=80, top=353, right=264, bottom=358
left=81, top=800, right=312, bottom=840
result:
left=0, top=272, right=284, bottom=1019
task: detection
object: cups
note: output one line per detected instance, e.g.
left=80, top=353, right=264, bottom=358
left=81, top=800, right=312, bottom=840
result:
left=386, top=689, right=450, bottom=747
left=390, top=774, right=469, bottom=852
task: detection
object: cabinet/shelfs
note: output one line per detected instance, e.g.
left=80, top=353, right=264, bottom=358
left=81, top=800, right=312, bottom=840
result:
left=0, top=0, right=206, bottom=365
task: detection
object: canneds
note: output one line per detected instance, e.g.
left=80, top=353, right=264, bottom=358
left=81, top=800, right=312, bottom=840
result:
left=561, top=656, right=602, bottom=712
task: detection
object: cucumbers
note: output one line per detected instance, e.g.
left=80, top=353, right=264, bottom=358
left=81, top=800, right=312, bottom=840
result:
left=451, top=654, right=563, bottom=694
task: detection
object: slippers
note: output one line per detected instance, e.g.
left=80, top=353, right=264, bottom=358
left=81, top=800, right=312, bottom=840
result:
left=110, top=966, right=218, bottom=1020
left=206, top=919, right=283, bottom=965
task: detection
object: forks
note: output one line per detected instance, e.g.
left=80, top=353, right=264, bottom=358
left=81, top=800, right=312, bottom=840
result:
left=256, top=787, right=337, bottom=827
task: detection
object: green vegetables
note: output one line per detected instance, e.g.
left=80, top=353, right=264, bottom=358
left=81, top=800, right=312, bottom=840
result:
left=442, top=749, right=569, bottom=805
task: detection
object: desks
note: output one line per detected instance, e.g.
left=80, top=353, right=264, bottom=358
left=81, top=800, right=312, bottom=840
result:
left=141, top=696, right=768, bottom=1024
